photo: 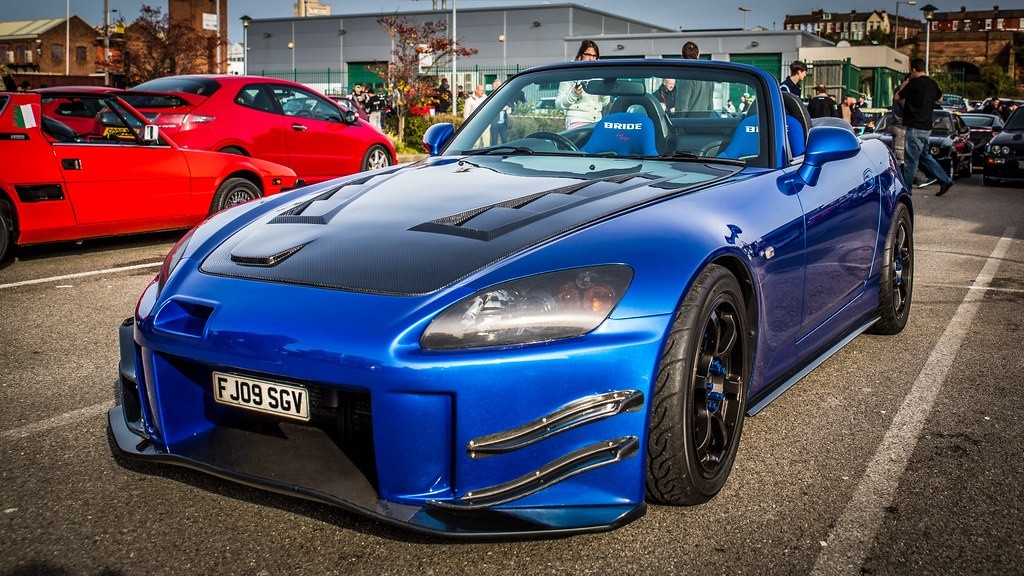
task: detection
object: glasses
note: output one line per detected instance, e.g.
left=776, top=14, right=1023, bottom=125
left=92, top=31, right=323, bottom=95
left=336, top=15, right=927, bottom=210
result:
left=582, top=52, right=597, bottom=58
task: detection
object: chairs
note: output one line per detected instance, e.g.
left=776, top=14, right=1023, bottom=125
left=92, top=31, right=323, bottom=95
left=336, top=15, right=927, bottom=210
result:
left=251, top=90, right=274, bottom=111
left=716, top=111, right=805, bottom=161
left=581, top=111, right=659, bottom=157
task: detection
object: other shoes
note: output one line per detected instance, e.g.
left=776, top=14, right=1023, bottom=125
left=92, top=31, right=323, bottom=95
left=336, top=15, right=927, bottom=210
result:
left=935, top=181, right=954, bottom=196
left=918, top=177, right=937, bottom=189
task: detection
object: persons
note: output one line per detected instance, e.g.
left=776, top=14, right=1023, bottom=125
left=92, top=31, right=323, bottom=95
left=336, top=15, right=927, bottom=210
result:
left=726, top=92, right=756, bottom=114
left=831, top=95, right=875, bottom=133
left=674, top=42, right=715, bottom=112
left=351, top=77, right=465, bottom=117
left=780, top=61, right=807, bottom=98
left=895, top=58, right=954, bottom=197
left=555, top=39, right=610, bottom=130
left=807, top=85, right=835, bottom=118
left=983, top=96, right=1019, bottom=122
left=891, top=73, right=938, bottom=187
left=463, top=84, right=491, bottom=150
left=488, top=79, right=515, bottom=146
left=652, top=78, right=676, bottom=112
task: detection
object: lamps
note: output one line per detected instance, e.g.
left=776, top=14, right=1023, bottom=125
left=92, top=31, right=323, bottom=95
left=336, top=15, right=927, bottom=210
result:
left=340, top=30, right=345, bottom=34
left=617, top=45, right=623, bottom=49
left=752, top=42, right=757, bottom=46
left=534, top=22, right=540, bottom=26
left=441, top=26, right=446, bottom=29
left=264, top=33, right=270, bottom=37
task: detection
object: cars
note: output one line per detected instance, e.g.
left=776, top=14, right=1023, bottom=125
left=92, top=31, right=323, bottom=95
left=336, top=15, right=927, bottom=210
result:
left=108, top=59, right=913, bottom=543
left=115, top=73, right=398, bottom=188
left=283, top=96, right=369, bottom=123
left=0, top=86, right=298, bottom=270
left=858, top=93, right=1024, bottom=186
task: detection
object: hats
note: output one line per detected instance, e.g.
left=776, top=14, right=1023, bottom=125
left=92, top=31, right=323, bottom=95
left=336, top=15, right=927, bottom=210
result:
left=813, top=85, right=826, bottom=92
left=366, top=90, right=374, bottom=93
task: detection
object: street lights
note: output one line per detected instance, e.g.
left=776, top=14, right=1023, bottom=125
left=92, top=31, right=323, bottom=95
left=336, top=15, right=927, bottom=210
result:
left=895, top=1, right=916, bottom=50
left=919, top=4, right=939, bottom=77
left=239, top=15, right=253, bottom=75
